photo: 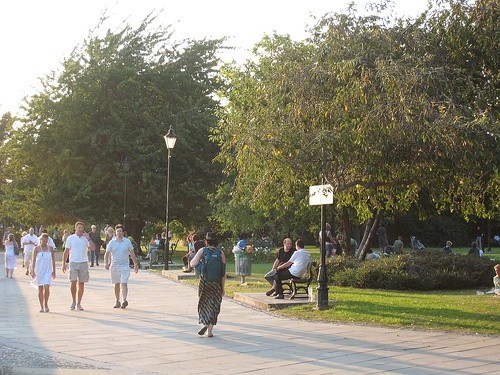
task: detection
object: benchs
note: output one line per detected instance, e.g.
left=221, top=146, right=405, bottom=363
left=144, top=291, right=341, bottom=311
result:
left=155, top=243, right=175, bottom=264
left=281, top=262, right=317, bottom=299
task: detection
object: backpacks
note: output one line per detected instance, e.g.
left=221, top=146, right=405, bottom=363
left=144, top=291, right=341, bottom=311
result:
left=200, top=247, right=226, bottom=281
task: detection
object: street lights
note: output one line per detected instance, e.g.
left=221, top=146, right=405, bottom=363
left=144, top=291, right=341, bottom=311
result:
left=159, top=124, right=178, bottom=271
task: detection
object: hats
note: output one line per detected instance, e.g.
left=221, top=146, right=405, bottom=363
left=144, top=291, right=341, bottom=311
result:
left=204, top=232, right=218, bottom=240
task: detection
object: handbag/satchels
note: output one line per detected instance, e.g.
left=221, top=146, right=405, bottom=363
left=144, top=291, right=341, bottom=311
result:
left=13, top=241, right=20, bottom=256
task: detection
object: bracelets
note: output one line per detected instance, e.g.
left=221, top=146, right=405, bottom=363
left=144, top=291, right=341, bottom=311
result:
left=88, top=238, right=91, bottom=241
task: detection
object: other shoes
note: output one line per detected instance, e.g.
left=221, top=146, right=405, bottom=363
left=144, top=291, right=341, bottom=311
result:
left=121, top=300, right=128, bottom=309
left=198, top=327, right=214, bottom=337
left=114, top=300, right=121, bottom=308
left=77, top=304, right=84, bottom=310
left=45, top=306, right=49, bottom=312
left=266, top=288, right=275, bottom=295
left=40, top=307, right=44, bottom=313
left=272, top=291, right=284, bottom=299
left=70, top=303, right=76, bottom=310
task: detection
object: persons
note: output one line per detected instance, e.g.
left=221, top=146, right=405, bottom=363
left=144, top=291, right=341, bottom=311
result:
left=443, top=241, right=455, bottom=255
left=467, top=242, right=479, bottom=256
left=376, top=225, right=388, bottom=253
left=62, top=222, right=97, bottom=310
left=318, top=223, right=335, bottom=257
left=145, top=234, right=160, bottom=262
left=104, top=224, right=139, bottom=309
left=104, top=224, right=134, bottom=266
left=393, top=235, right=403, bottom=254
left=182, top=230, right=205, bottom=273
left=476, top=225, right=483, bottom=248
left=492, top=264, right=500, bottom=292
left=53, top=227, right=58, bottom=247
left=89, top=224, right=100, bottom=267
left=62, top=229, right=71, bottom=263
left=2, top=227, right=19, bottom=278
left=190, top=232, right=226, bottom=337
left=264, top=238, right=312, bottom=299
left=31, top=234, right=56, bottom=312
left=20, top=228, right=56, bottom=275
